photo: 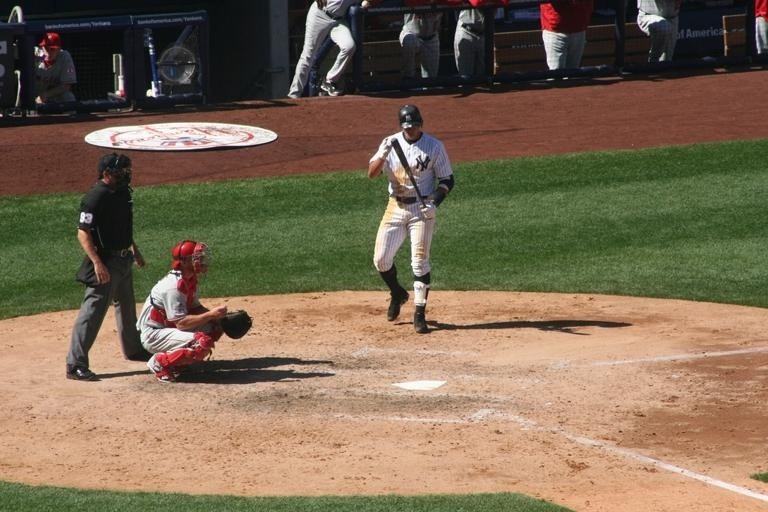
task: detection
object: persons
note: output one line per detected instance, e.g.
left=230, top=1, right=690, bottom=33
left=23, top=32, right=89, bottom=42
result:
left=366, top=104, right=457, bottom=334
left=32, top=32, right=77, bottom=107
left=637, top=1, right=680, bottom=69
left=134, top=238, right=255, bottom=383
left=449, top=2, right=497, bottom=88
left=392, top=2, right=440, bottom=81
left=62, top=151, right=155, bottom=382
left=288, top=1, right=359, bottom=99
left=539, top=2, right=594, bottom=80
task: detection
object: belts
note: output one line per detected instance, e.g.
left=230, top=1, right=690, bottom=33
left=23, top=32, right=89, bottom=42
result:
left=323, top=9, right=343, bottom=20
left=396, top=196, right=416, bottom=204
left=110, top=249, right=128, bottom=257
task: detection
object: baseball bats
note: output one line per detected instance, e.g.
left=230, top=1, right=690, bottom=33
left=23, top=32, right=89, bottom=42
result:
left=390, top=138, right=425, bottom=208
left=148, top=36, right=161, bottom=108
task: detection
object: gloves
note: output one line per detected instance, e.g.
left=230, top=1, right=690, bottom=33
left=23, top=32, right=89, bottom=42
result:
left=420, top=204, right=435, bottom=219
left=380, top=139, right=393, bottom=160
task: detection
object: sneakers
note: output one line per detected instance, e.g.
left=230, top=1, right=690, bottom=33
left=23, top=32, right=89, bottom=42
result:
left=414, top=306, right=428, bottom=333
left=147, top=356, right=175, bottom=381
left=387, top=288, right=409, bottom=320
left=320, top=82, right=341, bottom=96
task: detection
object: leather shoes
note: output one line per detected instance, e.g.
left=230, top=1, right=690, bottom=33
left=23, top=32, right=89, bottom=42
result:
left=67, top=364, right=95, bottom=380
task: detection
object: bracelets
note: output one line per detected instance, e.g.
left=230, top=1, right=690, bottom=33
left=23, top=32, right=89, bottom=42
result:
left=378, top=152, right=388, bottom=161
left=430, top=187, right=449, bottom=205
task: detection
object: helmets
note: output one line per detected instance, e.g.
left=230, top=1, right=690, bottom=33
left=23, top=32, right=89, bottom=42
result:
left=172, top=241, right=208, bottom=273
left=399, top=105, right=422, bottom=128
left=97, top=153, right=132, bottom=179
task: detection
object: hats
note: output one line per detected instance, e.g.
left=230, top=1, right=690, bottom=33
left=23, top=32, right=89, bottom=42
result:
left=40, top=32, right=60, bottom=49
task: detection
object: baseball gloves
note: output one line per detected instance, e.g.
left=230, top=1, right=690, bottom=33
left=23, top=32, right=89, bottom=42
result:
left=218, top=311, right=251, bottom=339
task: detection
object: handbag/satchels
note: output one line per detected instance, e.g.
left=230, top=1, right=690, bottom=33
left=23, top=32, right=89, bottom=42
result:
left=77, top=254, right=107, bottom=287
